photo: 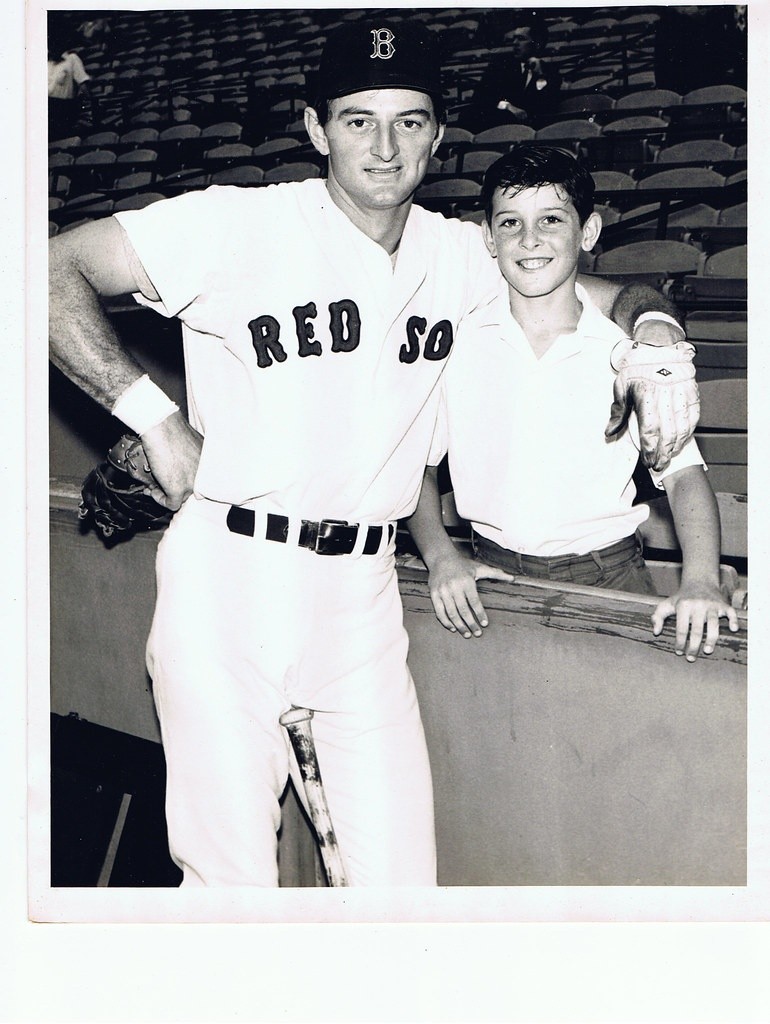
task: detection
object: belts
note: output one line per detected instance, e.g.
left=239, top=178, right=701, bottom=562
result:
left=228, top=506, right=393, bottom=557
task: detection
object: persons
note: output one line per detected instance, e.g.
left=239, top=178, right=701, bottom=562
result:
left=456, top=18, right=563, bottom=124
left=405, top=148, right=740, bottom=663
left=49, top=20, right=700, bottom=886
left=654, top=6, right=747, bottom=94
left=48, top=31, right=90, bottom=136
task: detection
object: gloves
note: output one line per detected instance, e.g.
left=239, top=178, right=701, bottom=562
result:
left=604, top=338, right=702, bottom=472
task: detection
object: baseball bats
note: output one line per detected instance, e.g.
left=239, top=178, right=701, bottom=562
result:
left=277, top=706, right=348, bottom=887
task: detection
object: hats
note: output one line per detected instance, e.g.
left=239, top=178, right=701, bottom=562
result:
left=318, top=17, right=448, bottom=131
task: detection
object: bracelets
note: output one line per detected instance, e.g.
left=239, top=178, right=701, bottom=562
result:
left=632, top=311, right=686, bottom=338
left=110, top=373, right=180, bottom=438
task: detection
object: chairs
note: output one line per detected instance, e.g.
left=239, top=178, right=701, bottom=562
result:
left=48, top=6, right=748, bottom=618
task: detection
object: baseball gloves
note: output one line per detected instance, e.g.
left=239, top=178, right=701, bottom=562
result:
left=75, top=433, right=175, bottom=548
